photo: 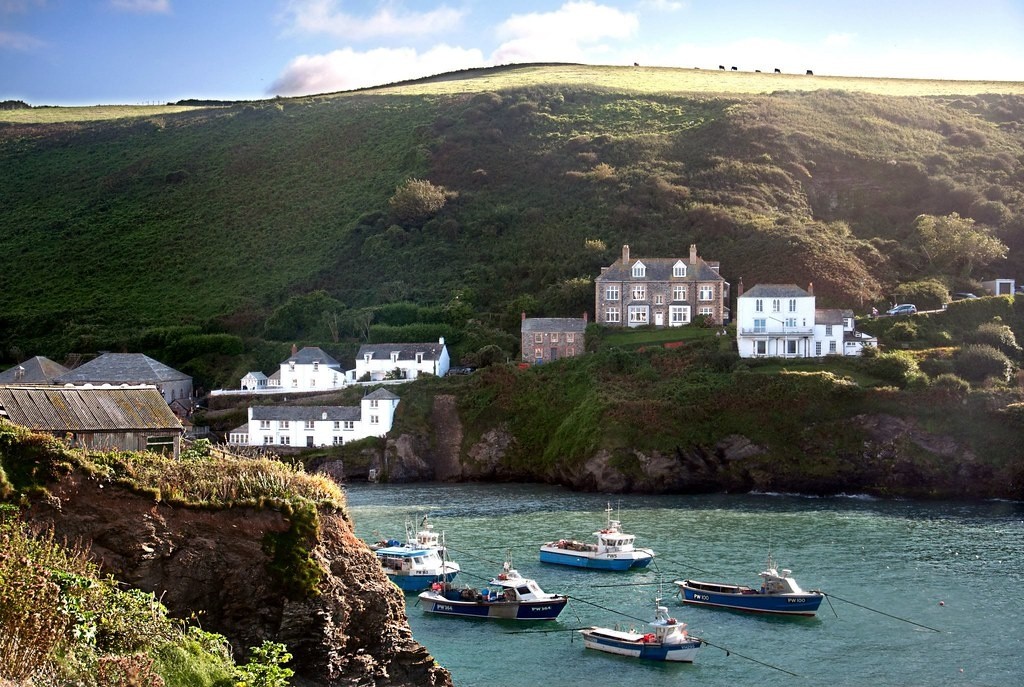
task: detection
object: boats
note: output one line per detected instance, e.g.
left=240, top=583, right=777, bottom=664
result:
left=368, top=512, right=460, bottom=594
left=674, top=566, right=825, bottom=620
left=418, top=529, right=569, bottom=620
left=578, top=589, right=702, bottom=663
left=539, top=500, right=656, bottom=571
